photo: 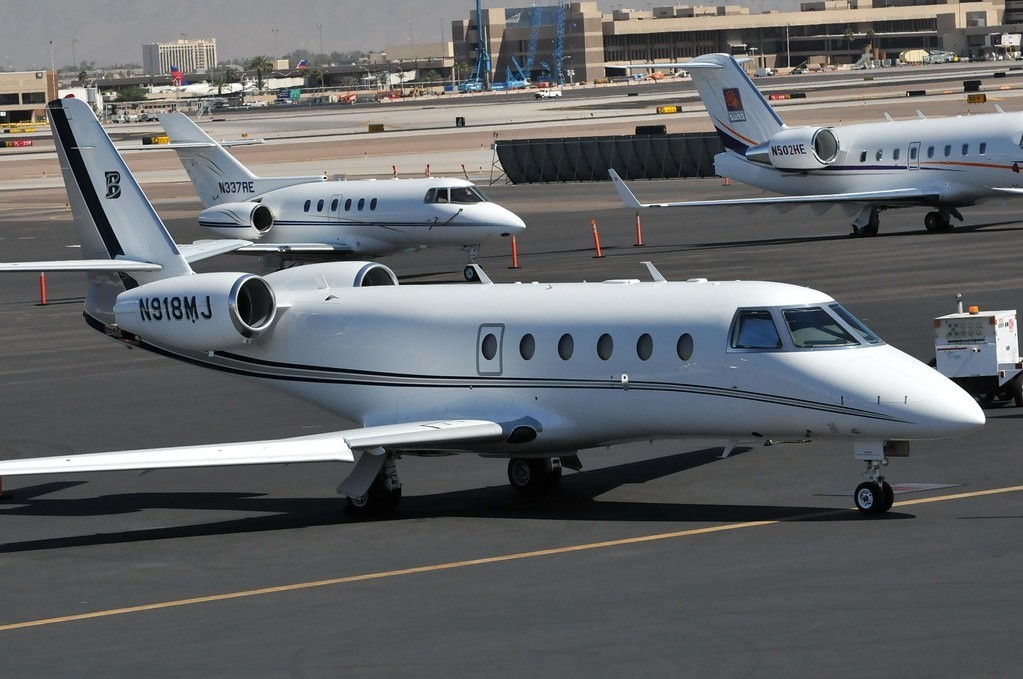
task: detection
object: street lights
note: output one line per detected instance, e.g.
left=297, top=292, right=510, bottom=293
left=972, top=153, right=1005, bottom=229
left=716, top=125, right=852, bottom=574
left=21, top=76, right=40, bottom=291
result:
left=438, top=15, right=445, bottom=58
left=271, top=69, right=300, bottom=99
left=316, top=23, right=325, bottom=66
left=358, top=66, right=371, bottom=100
left=47, top=39, right=57, bottom=100
left=559, top=55, right=571, bottom=86
left=786, top=23, right=791, bottom=70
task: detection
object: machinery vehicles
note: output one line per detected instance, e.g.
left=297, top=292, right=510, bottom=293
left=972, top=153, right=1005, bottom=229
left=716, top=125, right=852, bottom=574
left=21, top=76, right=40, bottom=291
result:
left=535, top=89, right=563, bottom=100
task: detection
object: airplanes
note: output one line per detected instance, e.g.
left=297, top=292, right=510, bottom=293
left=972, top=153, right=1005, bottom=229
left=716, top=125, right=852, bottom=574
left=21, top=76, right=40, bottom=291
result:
left=610, top=52, right=1019, bottom=235
left=145, top=65, right=246, bottom=117
left=0, top=98, right=986, bottom=515
left=110, top=113, right=526, bottom=265
